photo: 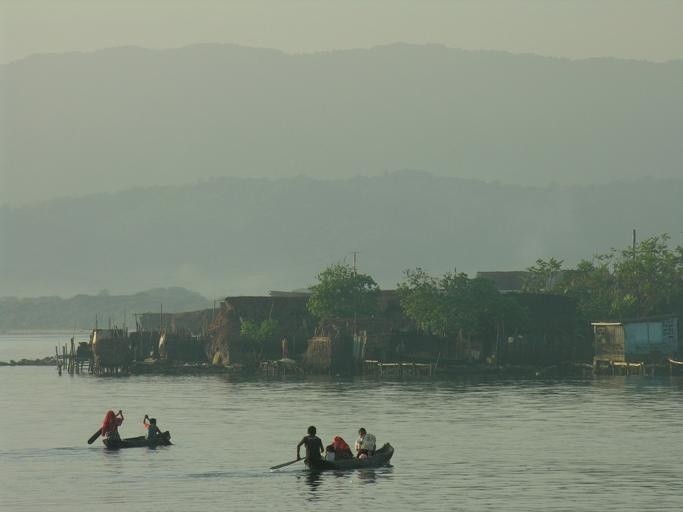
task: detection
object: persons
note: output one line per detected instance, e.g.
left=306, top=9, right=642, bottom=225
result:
left=101, top=409, right=124, bottom=440
left=296, top=425, right=325, bottom=461
left=356, top=427, right=376, bottom=457
left=326, top=436, right=353, bottom=461
left=141, top=414, right=159, bottom=441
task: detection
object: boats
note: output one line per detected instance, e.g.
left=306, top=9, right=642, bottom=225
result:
left=304, top=441, right=395, bottom=471
left=103, top=430, right=172, bottom=447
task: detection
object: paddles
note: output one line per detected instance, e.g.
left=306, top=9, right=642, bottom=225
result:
left=146, top=416, right=172, bottom=444
left=269, top=452, right=321, bottom=469
left=87, top=412, right=119, bottom=444
